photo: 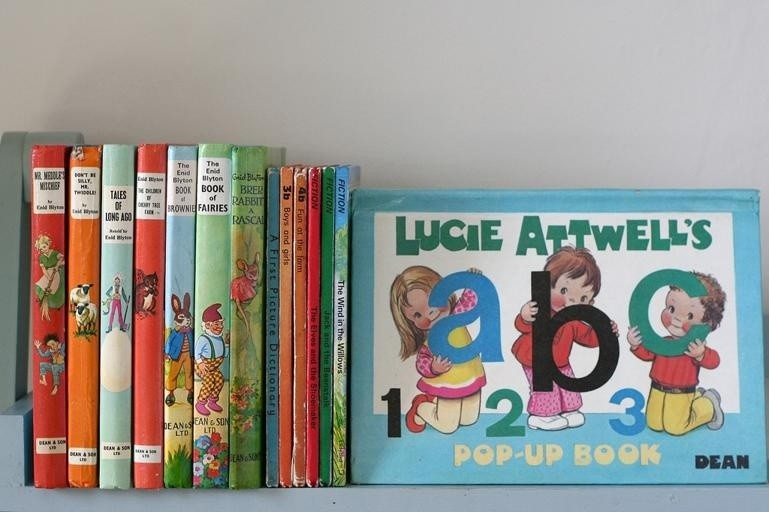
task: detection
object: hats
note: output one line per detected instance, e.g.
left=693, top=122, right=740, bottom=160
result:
left=201, top=303, right=222, bottom=323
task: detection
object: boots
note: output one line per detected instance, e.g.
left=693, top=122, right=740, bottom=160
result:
left=165, top=394, right=175, bottom=407
left=195, top=400, right=210, bottom=415
left=209, top=398, right=223, bottom=411
left=187, top=393, right=193, bottom=405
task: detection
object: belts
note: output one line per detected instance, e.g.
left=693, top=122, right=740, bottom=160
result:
left=651, top=381, right=695, bottom=395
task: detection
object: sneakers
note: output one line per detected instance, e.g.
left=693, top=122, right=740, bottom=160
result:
left=105, top=328, right=127, bottom=333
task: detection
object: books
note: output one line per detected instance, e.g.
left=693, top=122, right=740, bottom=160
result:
left=344, top=185, right=767, bottom=488
left=27, top=142, right=361, bottom=489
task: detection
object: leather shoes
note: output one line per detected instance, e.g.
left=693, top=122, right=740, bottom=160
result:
left=405, top=393, right=437, bottom=433
left=560, top=409, right=586, bottom=429
left=697, top=387, right=724, bottom=430
left=527, top=414, right=568, bottom=431
